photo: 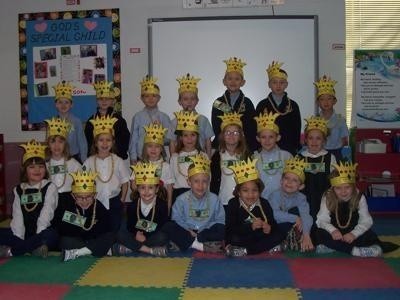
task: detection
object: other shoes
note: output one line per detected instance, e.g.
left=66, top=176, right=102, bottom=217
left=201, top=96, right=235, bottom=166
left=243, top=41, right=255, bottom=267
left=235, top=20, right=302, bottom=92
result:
left=360, top=244, right=381, bottom=258
left=1, top=247, right=13, bottom=260
left=315, top=245, right=336, bottom=254
left=116, top=244, right=134, bottom=255
left=62, top=249, right=79, bottom=261
left=224, top=244, right=247, bottom=258
left=152, top=246, right=167, bottom=258
left=269, top=243, right=289, bottom=255
left=203, top=243, right=223, bottom=253
left=32, top=245, right=47, bottom=258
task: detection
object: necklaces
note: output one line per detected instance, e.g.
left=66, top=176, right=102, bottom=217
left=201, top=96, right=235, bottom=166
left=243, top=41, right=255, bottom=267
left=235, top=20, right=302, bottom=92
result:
left=136, top=196, right=156, bottom=227
left=47, top=158, right=68, bottom=189
left=22, top=180, right=43, bottom=211
left=181, top=190, right=212, bottom=220
left=73, top=200, right=98, bottom=232
left=258, top=150, right=284, bottom=176
left=175, top=153, right=189, bottom=177
left=92, top=153, right=115, bottom=183
left=220, top=89, right=245, bottom=116
left=333, top=198, right=354, bottom=230
left=304, top=155, right=325, bottom=176
left=268, top=95, right=292, bottom=118
left=241, top=198, right=269, bottom=225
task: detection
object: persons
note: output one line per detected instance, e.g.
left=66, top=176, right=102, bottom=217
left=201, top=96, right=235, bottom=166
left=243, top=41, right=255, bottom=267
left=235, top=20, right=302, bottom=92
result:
left=127, top=83, right=175, bottom=164
left=49, top=180, right=118, bottom=263
left=44, top=92, right=89, bottom=165
left=167, top=118, right=213, bottom=202
left=311, top=162, right=384, bottom=257
left=159, top=163, right=228, bottom=253
left=223, top=177, right=285, bottom=257
left=0, top=157, right=60, bottom=259
left=265, top=167, right=315, bottom=254
left=111, top=173, right=170, bottom=257
left=41, top=133, right=85, bottom=198
left=78, top=130, right=130, bottom=234
left=166, top=86, right=215, bottom=162
left=255, top=69, right=303, bottom=157
left=296, top=124, right=339, bottom=224
left=84, top=87, right=130, bottom=162
left=210, top=70, right=257, bottom=152
left=249, top=127, right=295, bottom=199
left=315, top=89, right=350, bottom=162
left=210, top=122, right=249, bottom=219
left=127, top=139, right=173, bottom=219
left=33, top=43, right=107, bottom=98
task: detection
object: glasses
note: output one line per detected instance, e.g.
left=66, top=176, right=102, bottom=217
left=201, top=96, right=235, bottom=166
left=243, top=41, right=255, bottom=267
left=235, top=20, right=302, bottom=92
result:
left=224, top=131, right=241, bottom=135
